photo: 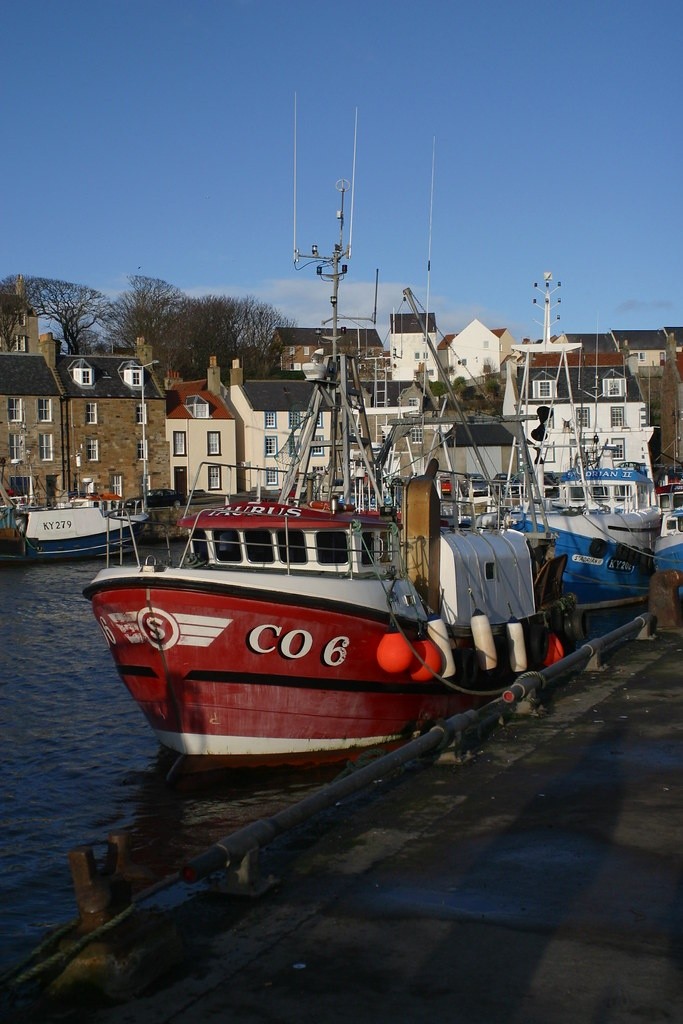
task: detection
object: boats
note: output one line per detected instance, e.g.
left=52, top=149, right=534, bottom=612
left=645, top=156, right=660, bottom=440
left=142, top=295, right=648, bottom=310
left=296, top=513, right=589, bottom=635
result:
left=0, top=455, right=150, bottom=566
left=82, top=88, right=683, bottom=794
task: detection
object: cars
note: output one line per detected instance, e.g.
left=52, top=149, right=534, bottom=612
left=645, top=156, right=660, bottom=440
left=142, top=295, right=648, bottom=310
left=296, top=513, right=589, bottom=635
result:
left=125, top=488, right=186, bottom=511
left=439, top=473, right=451, bottom=496
left=459, top=472, right=492, bottom=498
left=492, top=472, right=523, bottom=495
